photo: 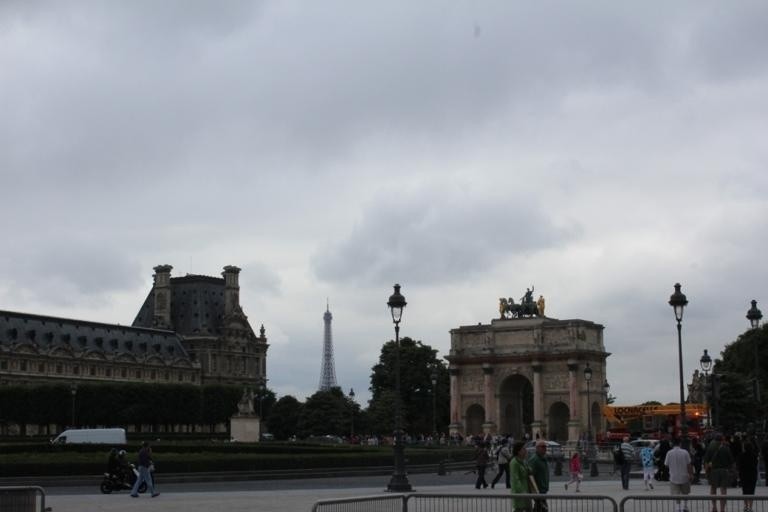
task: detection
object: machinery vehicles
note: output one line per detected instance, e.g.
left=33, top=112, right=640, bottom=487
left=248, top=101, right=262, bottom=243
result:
left=597, top=402, right=719, bottom=463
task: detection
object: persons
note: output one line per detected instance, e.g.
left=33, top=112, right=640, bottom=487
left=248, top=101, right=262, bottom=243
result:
left=538, top=296, right=545, bottom=317
left=107, top=441, right=160, bottom=497
left=349, top=432, right=767, bottom=512
left=520, top=285, right=534, bottom=303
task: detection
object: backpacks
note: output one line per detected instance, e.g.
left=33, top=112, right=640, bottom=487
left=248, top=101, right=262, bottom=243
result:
left=613, top=444, right=625, bottom=465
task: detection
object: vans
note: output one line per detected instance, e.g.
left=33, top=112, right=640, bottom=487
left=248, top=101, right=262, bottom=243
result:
left=48, top=426, right=129, bottom=445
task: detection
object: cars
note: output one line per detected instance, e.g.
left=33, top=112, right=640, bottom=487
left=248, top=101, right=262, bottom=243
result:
left=623, top=438, right=662, bottom=463
left=261, top=429, right=394, bottom=448
left=521, top=440, right=563, bottom=461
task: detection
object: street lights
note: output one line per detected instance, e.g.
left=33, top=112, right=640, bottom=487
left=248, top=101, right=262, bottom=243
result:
left=70, top=378, right=78, bottom=429
left=747, top=298, right=764, bottom=480
left=583, top=359, right=599, bottom=478
left=667, top=281, right=690, bottom=452
left=699, top=348, right=713, bottom=471
left=387, top=282, right=412, bottom=493
left=429, top=366, right=439, bottom=448
left=602, top=379, right=611, bottom=432
left=348, top=388, right=357, bottom=442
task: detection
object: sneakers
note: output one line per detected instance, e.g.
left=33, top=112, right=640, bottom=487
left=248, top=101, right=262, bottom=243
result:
left=151, top=492, right=161, bottom=498
left=130, top=493, right=140, bottom=498
left=645, top=481, right=655, bottom=491
left=564, top=483, right=581, bottom=493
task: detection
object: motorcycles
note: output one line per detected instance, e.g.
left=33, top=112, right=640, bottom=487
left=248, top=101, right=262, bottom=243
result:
left=100, top=461, right=148, bottom=496
